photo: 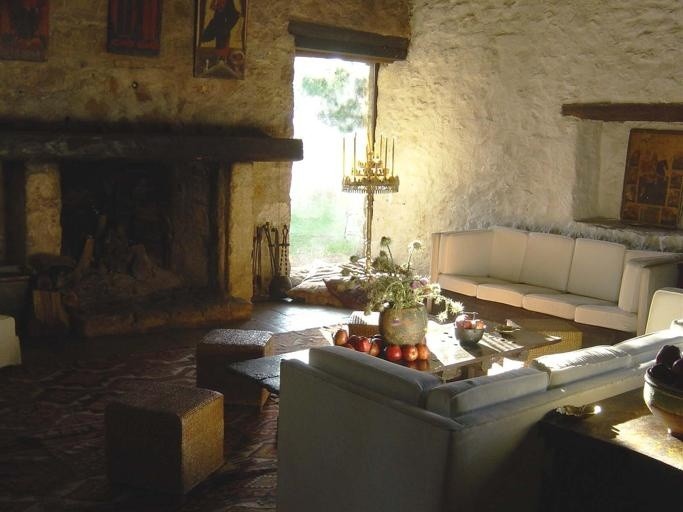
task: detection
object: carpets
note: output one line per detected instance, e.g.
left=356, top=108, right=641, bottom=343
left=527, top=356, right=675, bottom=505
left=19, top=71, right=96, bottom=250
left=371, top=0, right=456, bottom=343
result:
left=0, top=324, right=362, bottom=511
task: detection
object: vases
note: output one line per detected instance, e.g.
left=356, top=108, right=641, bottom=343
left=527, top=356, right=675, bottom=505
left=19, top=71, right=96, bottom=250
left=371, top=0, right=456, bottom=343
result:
left=377, top=303, right=429, bottom=348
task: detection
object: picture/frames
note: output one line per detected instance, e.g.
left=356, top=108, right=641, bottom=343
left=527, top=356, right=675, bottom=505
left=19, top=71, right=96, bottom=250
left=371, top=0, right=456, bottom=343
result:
left=104, top=0, right=164, bottom=59
left=0, top=0, right=49, bottom=63
left=193, top=0, right=247, bottom=81
left=618, top=127, right=682, bottom=228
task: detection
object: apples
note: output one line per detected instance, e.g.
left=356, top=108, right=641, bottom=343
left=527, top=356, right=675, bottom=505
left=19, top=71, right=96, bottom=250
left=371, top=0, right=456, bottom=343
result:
left=455, top=314, right=484, bottom=330
left=331, top=329, right=430, bottom=362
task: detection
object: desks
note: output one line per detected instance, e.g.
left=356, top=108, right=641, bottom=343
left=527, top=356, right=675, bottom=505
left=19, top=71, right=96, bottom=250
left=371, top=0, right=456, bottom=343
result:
left=544, top=378, right=682, bottom=473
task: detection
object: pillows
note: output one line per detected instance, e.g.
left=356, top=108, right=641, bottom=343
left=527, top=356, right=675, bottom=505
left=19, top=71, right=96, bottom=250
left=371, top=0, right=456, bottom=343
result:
left=320, top=276, right=369, bottom=310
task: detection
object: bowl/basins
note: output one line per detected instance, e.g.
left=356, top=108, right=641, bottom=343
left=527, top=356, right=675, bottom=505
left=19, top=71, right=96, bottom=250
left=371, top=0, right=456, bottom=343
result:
left=641, top=368, right=683, bottom=438
left=454, top=326, right=485, bottom=344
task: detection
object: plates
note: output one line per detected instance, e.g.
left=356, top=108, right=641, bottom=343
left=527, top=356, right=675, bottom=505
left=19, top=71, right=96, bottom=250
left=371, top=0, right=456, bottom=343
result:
left=494, top=325, right=520, bottom=334
left=555, top=403, right=602, bottom=420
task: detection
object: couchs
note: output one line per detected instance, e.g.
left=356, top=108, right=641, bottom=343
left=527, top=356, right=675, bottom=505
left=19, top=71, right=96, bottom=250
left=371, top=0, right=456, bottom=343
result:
left=274, top=286, right=681, bottom=511
left=427, top=225, right=682, bottom=336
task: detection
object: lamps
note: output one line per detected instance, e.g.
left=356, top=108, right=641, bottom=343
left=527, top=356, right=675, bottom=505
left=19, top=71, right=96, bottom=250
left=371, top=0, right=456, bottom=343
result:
left=337, top=124, right=402, bottom=318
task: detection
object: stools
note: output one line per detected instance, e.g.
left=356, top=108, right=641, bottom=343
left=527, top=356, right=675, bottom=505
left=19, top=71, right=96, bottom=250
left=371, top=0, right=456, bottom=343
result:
left=102, top=378, right=226, bottom=498
left=516, top=317, right=583, bottom=369
left=192, top=327, right=277, bottom=409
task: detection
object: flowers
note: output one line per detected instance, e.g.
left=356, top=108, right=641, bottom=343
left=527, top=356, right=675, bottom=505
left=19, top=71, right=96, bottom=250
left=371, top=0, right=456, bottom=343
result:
left=333, top=232, right=471, bottom=317
